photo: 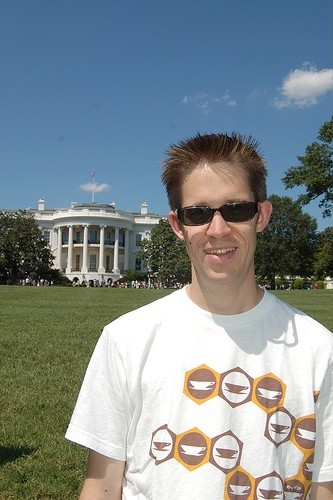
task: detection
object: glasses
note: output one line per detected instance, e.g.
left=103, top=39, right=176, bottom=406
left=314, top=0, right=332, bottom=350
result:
left=175, top=202, right=259, bottom=226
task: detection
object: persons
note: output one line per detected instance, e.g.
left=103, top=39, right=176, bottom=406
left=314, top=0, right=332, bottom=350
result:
left=72, top=274, right=190, bottom=289
left=260, top=279, right=319, bottom=292
left=19, top=274, right=54, bottom=286
left=65, top=130, right=333, bottom=500
